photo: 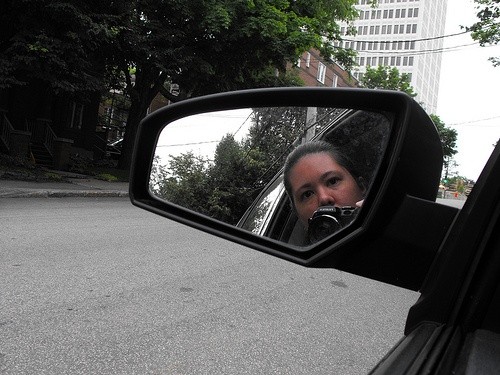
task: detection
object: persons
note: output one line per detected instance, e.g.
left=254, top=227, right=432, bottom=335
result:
left=282, top=140, right=369, bottom=226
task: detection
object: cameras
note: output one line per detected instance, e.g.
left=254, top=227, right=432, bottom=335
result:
left=308, top=206, right=361, bottom=245
